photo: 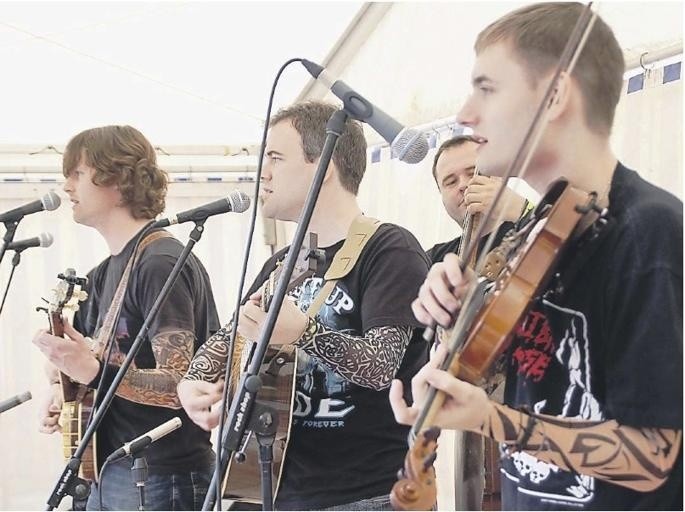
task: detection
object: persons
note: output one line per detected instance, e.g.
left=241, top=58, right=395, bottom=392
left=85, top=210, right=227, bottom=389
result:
left=29, top=125, right=220, bottom=512
left=419, top=132, right=536, bottom=302
left=388, top=1, right=683, bottom=508
left=174, top=100, right=440, bottom=509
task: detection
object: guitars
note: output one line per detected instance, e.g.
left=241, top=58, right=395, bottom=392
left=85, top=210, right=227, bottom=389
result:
left=35, top=265, right=99, bottom=486
left=221, top=233, right=325, bottom=507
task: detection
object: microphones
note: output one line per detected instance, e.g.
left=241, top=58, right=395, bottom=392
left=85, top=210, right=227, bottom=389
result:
left=121, top=416, right=182, bottom=455
left=0, top=192, right=61, bottom=221
left=6, top=231, right=54, bottom=250
left=167, top=190, right=250, bottom=226
left=0, top=390, right=32, bottom=413
left=317, top=69, right=428, bottom=165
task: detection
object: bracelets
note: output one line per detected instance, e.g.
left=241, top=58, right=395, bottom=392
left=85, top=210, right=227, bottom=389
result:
left=521, top=196, right=534, bottom=220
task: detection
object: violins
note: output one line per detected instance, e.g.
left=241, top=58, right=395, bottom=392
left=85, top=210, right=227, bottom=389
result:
left=390, top=178, right=609, bottom=511
